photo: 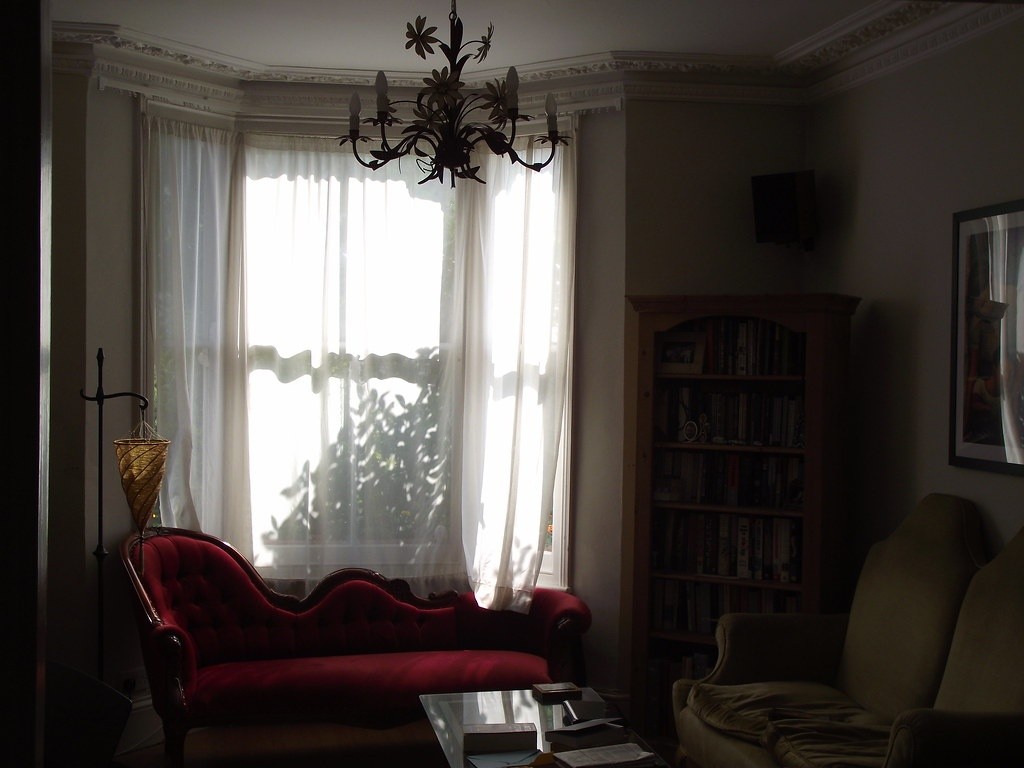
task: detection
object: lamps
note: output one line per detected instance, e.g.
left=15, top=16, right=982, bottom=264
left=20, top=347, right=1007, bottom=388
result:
left=334, top=0, right=572, bottom=189
left=80, top=347, right=172, bottom=714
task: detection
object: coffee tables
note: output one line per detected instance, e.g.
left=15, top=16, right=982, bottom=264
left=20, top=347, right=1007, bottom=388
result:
left=418, top=688, right=673, bottom=768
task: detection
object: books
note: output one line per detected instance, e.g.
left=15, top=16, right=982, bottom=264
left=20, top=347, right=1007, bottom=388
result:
left=646, top=316, right=803, bottom=739
left=462, top=681, right=654, bottom=768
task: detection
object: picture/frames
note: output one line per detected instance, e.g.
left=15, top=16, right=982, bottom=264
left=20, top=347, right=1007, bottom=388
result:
left=948, top=200, right=1024, bottom=476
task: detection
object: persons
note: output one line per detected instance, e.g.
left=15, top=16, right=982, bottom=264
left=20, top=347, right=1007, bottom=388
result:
left=972, top=347, right=1000, bottom=423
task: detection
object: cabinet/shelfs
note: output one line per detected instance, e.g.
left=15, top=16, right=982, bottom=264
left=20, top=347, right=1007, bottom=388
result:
left=628, top=292, right=862, bottom=748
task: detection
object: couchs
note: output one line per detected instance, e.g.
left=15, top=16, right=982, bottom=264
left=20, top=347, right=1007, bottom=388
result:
left=672, top=492, right=1024, bottom=768
left=117, top=527, right=592, bottom=768
